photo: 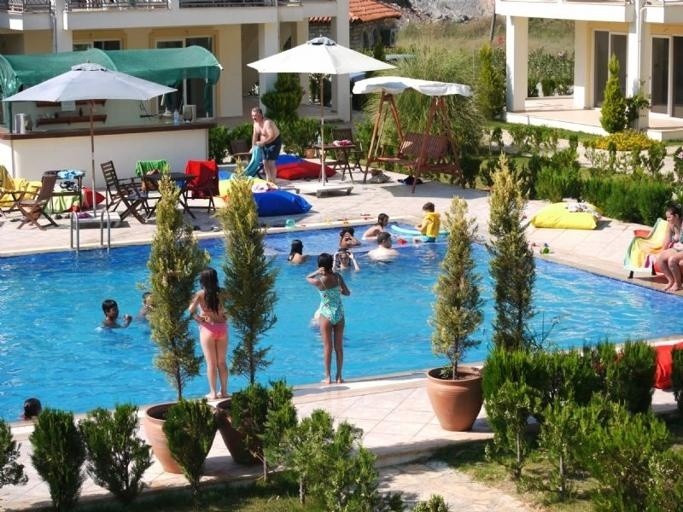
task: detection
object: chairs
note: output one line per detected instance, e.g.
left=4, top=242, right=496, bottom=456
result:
left=331, top=128, right=364, bottom=173
left=627, top=217, right=683, bottom=278
left=0, top=152, right=264, bottom=231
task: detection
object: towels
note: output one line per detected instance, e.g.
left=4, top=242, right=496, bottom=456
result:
left=623, top=218, right=669, bottom=271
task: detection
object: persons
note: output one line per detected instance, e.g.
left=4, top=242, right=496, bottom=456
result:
left=412, top=201, right=441, bottom=245
left=101, top=299, right=132, bottom=329
left=368, top=231, right=399, bottom=260
left=330, top=249, right=360, bottom=274
left=337, top=227, right=360, bottom=248
left=140, top=291, right=155, bottom=318
left=654, top=205, right=683, bottom=292
left=249, top=107, right=282, bottom=184
left=19, top=397, right=42, bottom=421
left=188, top=267, right=244, bottom=402
left=305, top=252, right=351, bottom=385
left=361, top=212, right=389, bottom=241
left=287, top=238, right=308, bottom=267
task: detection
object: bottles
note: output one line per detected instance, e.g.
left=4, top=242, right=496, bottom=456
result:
left=173, top=109, right=179, bottom=126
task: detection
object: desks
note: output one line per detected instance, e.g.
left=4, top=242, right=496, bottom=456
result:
left=312, top=142, right=356, bottom=182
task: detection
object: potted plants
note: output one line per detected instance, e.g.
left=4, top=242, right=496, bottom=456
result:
left=216, top=157, right=296, bottom=465
left=426, top=194, right=487, bottom=432
left=137, top=174, right=219, bottom=473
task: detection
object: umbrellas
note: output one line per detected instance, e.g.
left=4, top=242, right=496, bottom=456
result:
left=0, top=57, right=179, bottom=219
left=247, top=34, right=399, bottom=185
